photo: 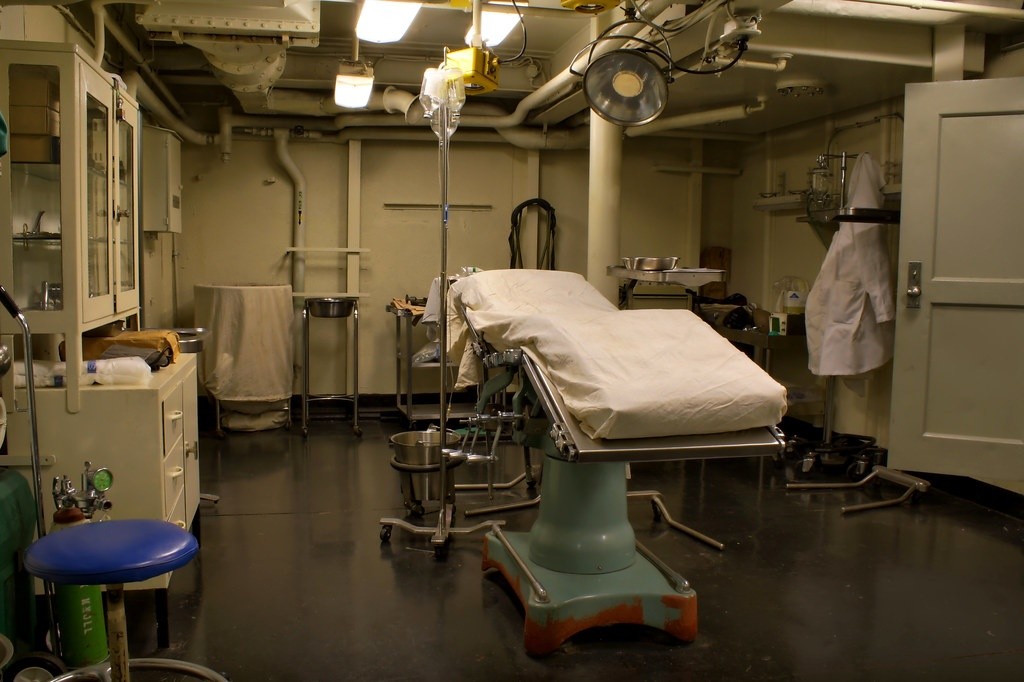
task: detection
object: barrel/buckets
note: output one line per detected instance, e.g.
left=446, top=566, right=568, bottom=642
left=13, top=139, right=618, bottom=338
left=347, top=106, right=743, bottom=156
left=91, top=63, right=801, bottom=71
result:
left=391, top=430, right=459, bottom=501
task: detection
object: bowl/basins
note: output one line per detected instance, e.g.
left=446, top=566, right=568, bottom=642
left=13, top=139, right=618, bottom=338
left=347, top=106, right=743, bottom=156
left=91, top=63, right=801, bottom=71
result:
left=622, top=256, right=681, bottom=271
left=305, top=297, right=356, bottom=318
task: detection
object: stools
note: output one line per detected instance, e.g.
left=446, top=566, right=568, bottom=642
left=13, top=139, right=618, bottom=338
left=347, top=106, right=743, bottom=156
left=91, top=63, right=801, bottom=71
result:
left=20, top=519, right=230, bottom=682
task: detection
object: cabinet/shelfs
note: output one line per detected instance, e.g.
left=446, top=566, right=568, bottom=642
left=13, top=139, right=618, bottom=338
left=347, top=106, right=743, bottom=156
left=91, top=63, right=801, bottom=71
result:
left=383, top=296, right=515, bottom=436
left=0, top=38, right=201, bottom=652
left=695, top=303, right=837, bottom=450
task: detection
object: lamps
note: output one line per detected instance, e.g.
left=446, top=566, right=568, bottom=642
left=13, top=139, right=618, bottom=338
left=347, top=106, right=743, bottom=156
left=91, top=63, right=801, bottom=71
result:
left=439, top=45, right=505, bottom=96
left=463, top=1, right=530, bottom=48
left=355, top=0, right=423, bottom=44
left=581, top=49, right=672, bottom=129
left=332, top=61, right=375, bottom=108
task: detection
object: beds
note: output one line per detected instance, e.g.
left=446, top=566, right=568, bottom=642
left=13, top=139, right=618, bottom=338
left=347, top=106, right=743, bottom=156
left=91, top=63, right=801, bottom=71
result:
left=448, top=268, right=794, bottom=662
